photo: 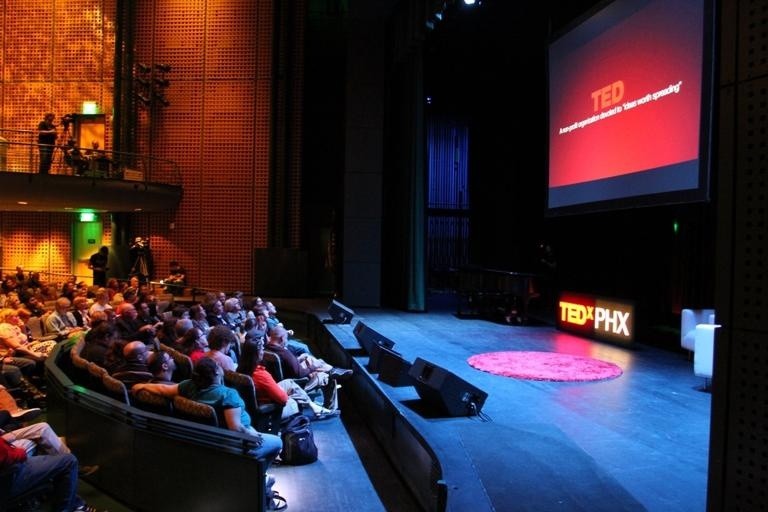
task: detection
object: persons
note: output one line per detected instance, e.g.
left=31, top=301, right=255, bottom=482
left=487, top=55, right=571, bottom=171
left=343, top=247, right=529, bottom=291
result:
left=36, top=112, right=124, bottom=176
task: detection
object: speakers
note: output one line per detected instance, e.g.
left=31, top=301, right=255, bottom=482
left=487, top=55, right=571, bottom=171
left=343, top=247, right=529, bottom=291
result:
left=408, top=357, right=489, bottom=414
left=368, top=338, right=401, bottom=373
left=327, top=299, right=355, bottom=324
left=353, top=320, right=396, bottom=355
left=377, top=346, right=415, bottom=386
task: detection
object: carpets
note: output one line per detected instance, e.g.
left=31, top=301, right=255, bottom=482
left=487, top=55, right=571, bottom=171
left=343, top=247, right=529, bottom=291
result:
left=467, top=349, right=624, bottom=384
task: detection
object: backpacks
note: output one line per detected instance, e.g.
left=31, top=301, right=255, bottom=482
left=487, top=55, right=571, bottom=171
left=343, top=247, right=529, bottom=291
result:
left=280, top=415, right=318, bottom=465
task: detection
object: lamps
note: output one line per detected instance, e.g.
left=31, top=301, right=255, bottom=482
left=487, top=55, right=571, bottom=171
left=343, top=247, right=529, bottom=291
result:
left=134, top=61, right=171, bottom=108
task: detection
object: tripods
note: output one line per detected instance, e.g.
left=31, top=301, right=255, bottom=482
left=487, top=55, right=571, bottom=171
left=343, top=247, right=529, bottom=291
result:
left=51, top=128, right=82, bottom=176
left=127, top=247, right=154, bottom=282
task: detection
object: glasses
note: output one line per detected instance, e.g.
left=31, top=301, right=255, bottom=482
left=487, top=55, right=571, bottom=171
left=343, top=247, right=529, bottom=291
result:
left=163, top=357, right=172, bottom=363
left=199, top=333, right=206, bottom=336
left=139, top=350, right=149, bottom=355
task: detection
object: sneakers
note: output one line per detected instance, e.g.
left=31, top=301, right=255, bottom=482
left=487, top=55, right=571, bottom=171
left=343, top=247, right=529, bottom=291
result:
left=314, top=404, right=342, bottom=419
left=265, top=472, right=276, bottom=483
left=79, top=465, right=98, bottom=479
left=73, top=505, right=112, bottom=512
left=9, top=407, right=41, bottom=422
left=331, top=366, right=353, bottom=378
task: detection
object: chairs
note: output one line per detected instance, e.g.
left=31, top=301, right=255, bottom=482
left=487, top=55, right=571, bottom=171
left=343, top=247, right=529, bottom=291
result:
left=681, top=306, right=715, bottom=390
left=17, top=292, right=309, bottom=512
